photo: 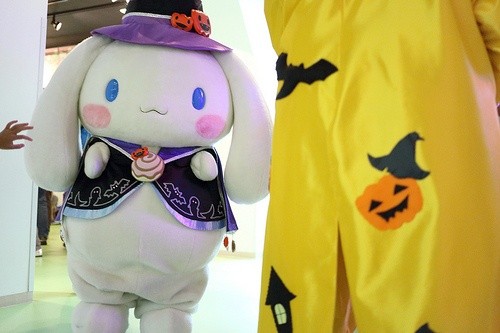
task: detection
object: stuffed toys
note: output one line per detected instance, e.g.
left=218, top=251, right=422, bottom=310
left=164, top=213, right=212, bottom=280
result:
left=21, top=0, right=276, bottom=333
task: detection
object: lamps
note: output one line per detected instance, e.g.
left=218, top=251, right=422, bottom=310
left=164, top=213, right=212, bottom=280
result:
left=51, top=13, right=63, bottom=30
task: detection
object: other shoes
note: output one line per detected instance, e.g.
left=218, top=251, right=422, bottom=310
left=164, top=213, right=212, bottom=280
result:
left=35, top=249, right=43, bottom=257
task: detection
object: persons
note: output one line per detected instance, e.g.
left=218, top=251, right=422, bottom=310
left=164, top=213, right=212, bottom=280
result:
left=34, top=187, right=65, bottom=258
left=0, top=120, right=34, bottom=149
left=256, top=1, right=500, bottom=333
